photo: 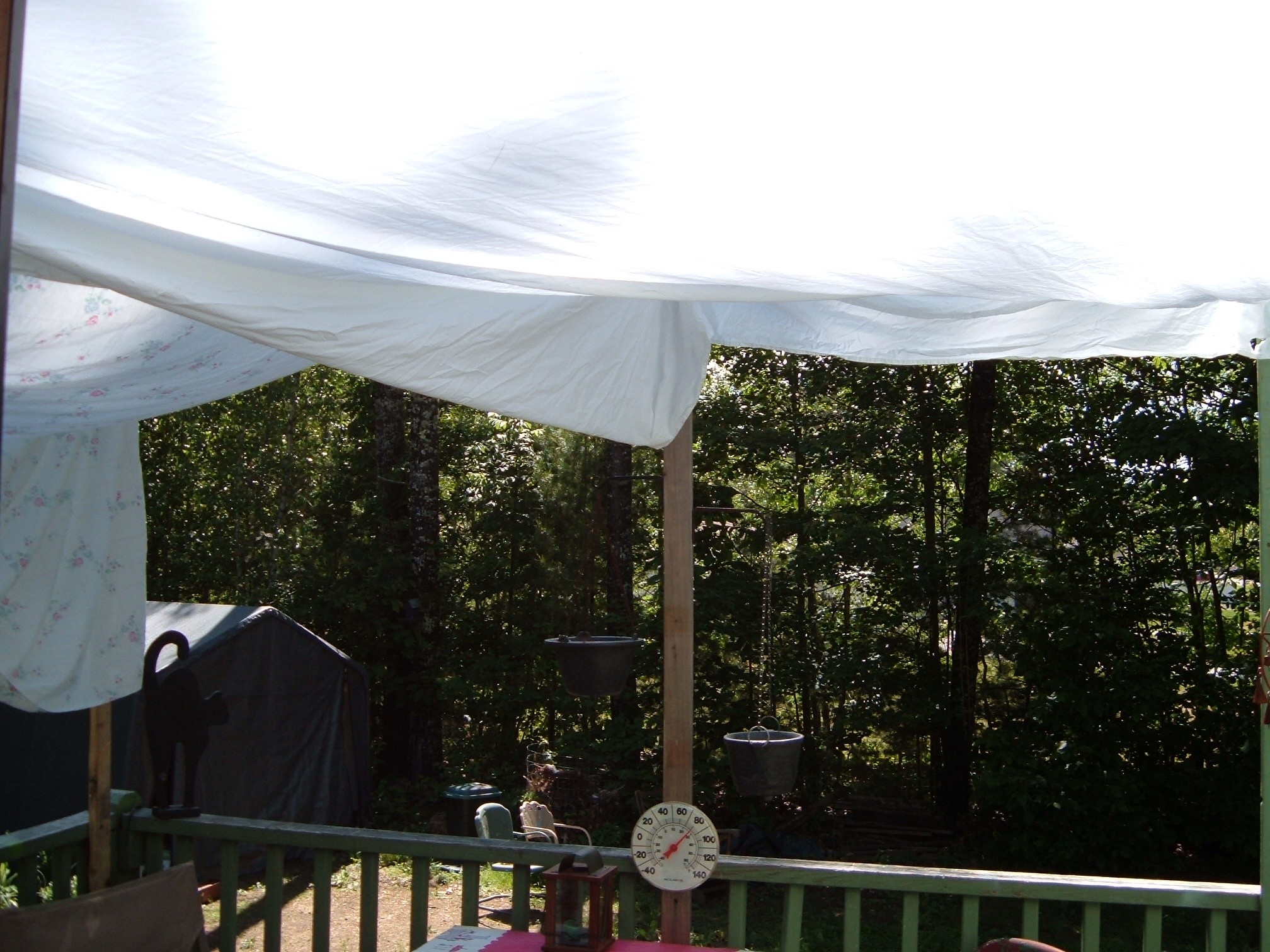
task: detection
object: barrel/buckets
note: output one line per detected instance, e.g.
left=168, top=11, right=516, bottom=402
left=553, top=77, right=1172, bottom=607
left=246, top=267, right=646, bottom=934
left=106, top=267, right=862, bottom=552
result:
left=722, top=730, right=805, bottom=796
left=544, top=631, right=645, bottom=696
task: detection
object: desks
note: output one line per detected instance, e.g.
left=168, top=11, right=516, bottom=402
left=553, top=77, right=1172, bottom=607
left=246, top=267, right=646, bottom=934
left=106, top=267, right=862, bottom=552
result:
left=411, top=926, right=545, bottom=952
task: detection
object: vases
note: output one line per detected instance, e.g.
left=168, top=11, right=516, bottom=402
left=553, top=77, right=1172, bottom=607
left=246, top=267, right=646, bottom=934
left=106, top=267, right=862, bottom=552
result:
left=724, top=729, right=804, bottom=795
left=544, top=631, right=642, bottom=696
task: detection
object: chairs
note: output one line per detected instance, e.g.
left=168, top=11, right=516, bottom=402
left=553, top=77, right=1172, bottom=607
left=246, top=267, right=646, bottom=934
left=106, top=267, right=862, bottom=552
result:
left=475, top=802, right=593, bottom=916
left=0, top=860, right=209, bottom=952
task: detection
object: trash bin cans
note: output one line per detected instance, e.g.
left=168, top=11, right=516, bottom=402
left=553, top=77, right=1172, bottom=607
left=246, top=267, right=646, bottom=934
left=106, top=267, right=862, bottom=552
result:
left=443, top=781, right=502, bottom=837
